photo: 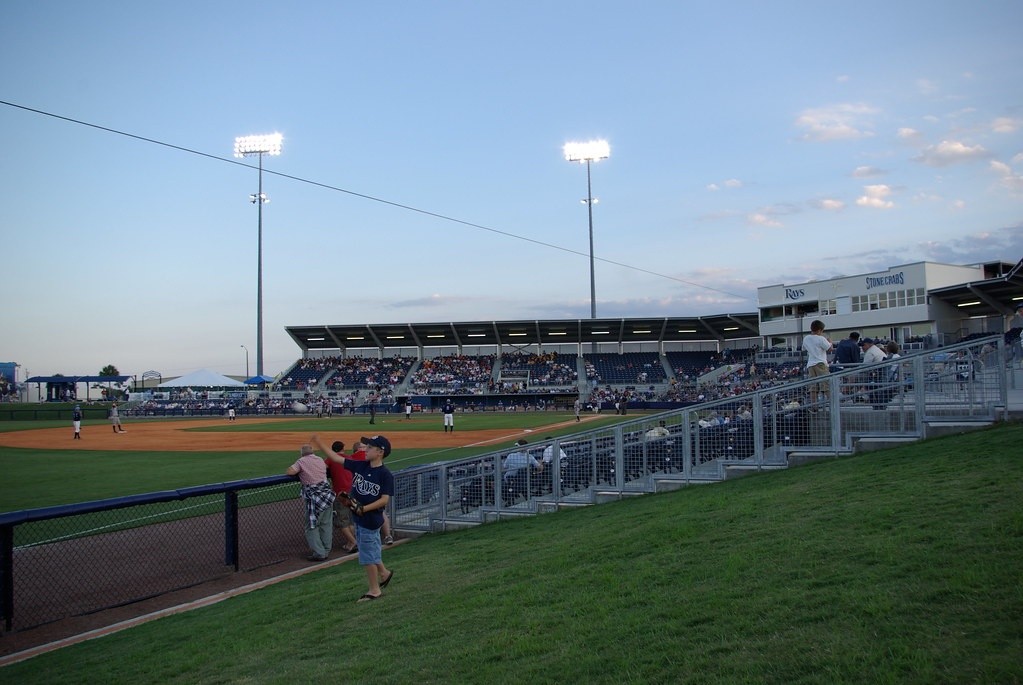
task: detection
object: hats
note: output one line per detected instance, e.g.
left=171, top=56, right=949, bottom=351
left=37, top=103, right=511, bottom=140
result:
left=332, top=441, right=345, bottom=453
left=360, top=435, right=391, bottom=459
left=857, top=337, right=873, bottom=347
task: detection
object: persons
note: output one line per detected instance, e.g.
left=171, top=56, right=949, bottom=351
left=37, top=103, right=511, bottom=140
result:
left=111, top=403, right=124, bottom=433
left=679, top=344, right=816, bottom=431
left=503, top=440, right=543, bottom=482
left=802, top=320, right=932, bottom=413
left=1, top=379, right=20, bottom=402
left=286, top=435, right=394, bottom=604
left=228, top=402, right=236, bottom=423
left=73, top=405, right=82, bottom=439
left=60, top=349, right=680, bottom=435
left=645, top=420, right=671, bottom=439
left=542, top=437, right=568, bottom=468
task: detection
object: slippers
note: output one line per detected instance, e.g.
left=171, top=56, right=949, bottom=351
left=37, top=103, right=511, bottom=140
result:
left=379, top=570, right=393, bottom=589
left=356, top=593, right=382, bottom=606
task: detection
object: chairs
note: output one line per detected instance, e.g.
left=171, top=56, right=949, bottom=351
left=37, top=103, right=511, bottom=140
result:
left=278, top=326, right=1022, bottom=513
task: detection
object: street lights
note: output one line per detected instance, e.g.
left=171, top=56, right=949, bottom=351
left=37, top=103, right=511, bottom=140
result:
left=233, top=133, right=284, bottom=372
left=564, top=138, right=611, bottom=350
left=240, top=345, right=249, bottom=383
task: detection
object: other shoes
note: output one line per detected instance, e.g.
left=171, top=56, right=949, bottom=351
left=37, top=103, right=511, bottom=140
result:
left=342, top=543, right=359, bottom=554
left=384, top=535, right=394, bottom=546
left=804, top=398, right=819, bottom=415
left=306, top=556, right=329, bottom=562
left=380, top=539, right=387, bottom=549
